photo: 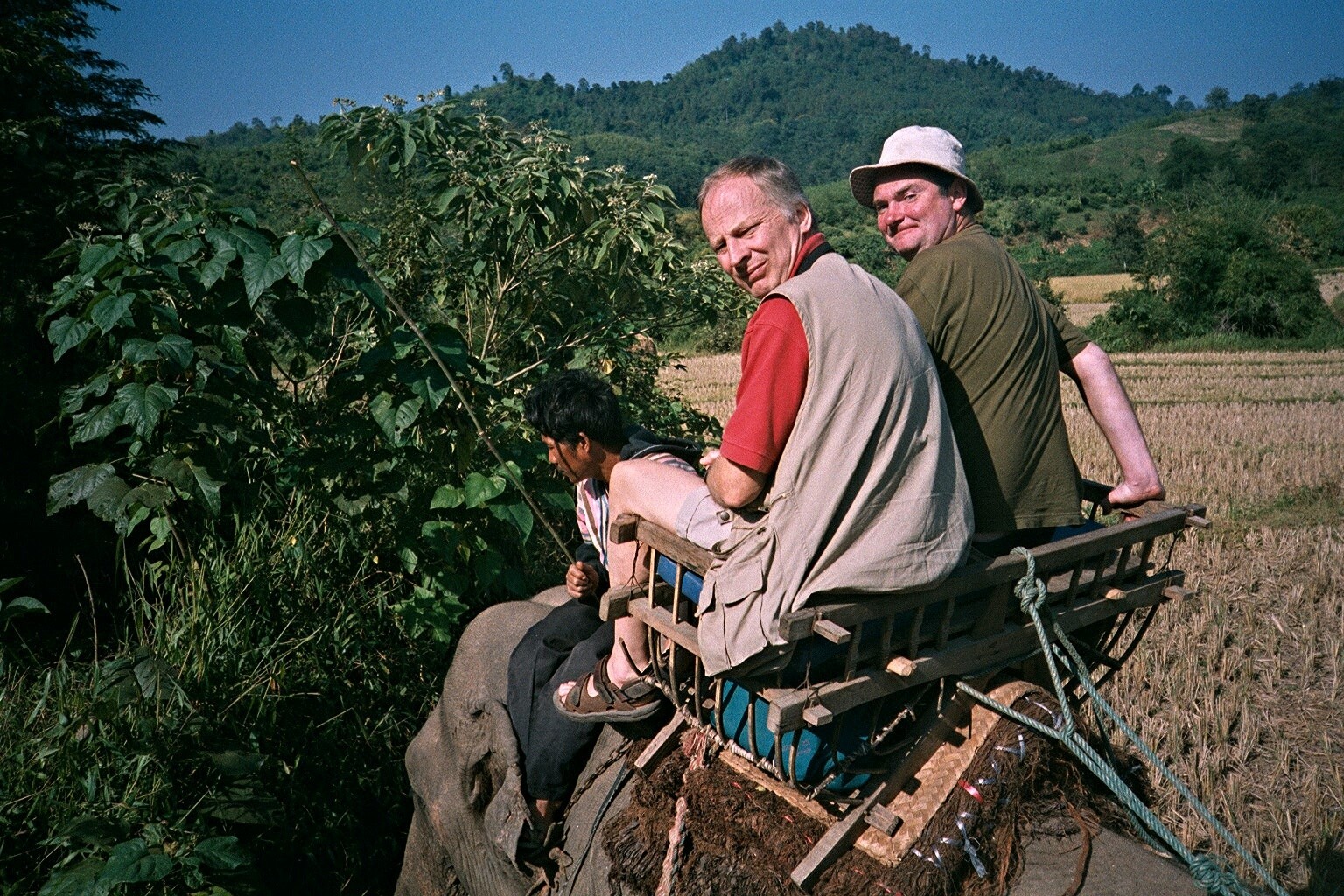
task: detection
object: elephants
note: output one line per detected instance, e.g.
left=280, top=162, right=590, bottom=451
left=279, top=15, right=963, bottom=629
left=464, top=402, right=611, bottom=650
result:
left=394, top=585, right=1231, bottom=896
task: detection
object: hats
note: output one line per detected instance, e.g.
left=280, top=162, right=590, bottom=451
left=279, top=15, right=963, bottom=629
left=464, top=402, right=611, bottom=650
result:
left=849, top=126, right=985, bottom=215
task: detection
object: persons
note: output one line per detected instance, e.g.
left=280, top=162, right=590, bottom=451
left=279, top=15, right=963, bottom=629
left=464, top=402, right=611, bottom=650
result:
left=553, top=156, right=974, bottom=722
left=849, top=125, right=1166, bottom=558
left=526, top=369, right=699, bottom=598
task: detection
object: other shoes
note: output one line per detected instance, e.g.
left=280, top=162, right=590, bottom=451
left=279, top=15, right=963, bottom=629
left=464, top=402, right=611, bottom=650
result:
left=517, top=830, right=546, bottom=849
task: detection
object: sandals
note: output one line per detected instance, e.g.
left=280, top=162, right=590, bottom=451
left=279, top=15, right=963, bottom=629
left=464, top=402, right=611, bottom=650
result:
left=550, top=654, right=663, bottom=722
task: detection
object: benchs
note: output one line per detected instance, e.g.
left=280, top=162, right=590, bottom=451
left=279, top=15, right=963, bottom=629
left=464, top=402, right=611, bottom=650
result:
left=597, top=480, right=1213, bottom=893
